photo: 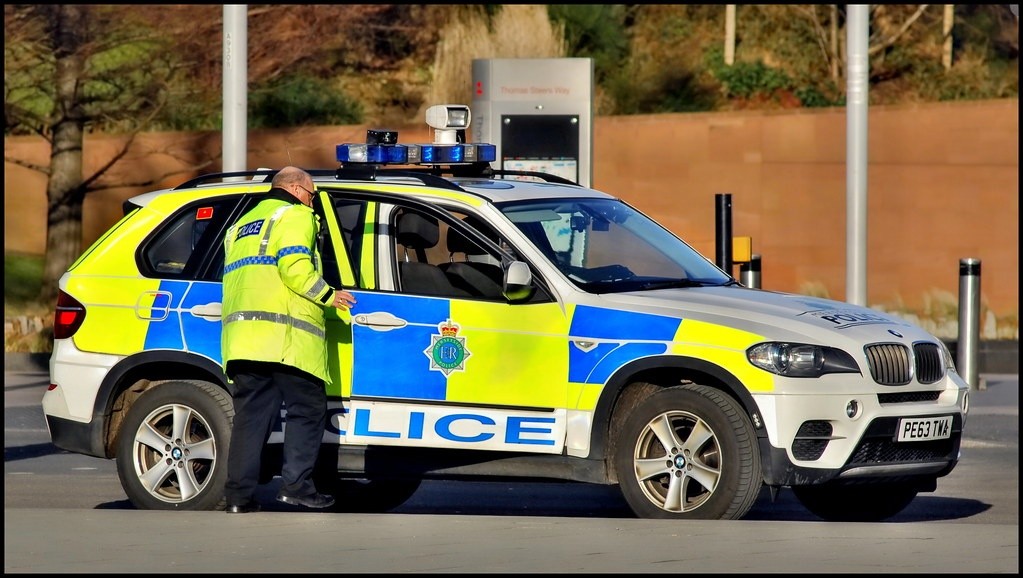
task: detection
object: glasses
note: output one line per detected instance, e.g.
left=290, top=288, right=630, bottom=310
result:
left=292, top=184, right=315, bottom=201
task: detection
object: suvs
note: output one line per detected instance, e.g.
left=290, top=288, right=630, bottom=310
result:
left=39, top=101, right=970, bottom=521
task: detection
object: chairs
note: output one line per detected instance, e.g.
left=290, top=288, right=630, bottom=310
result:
left=395, top=213, right=454, bottom=297
left=438, top=216, right=504, bottom=299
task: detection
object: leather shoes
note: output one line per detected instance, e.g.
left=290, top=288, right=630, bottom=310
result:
left=282, top=492, right=335, bottom=507
left=227, top=505, right=242, bottom=513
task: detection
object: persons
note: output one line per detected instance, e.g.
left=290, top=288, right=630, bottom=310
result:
left=221, top=167, right=357, bottom=513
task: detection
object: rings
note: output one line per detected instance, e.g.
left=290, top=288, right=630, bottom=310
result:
left=340, top=298, right=342, bottom=302
